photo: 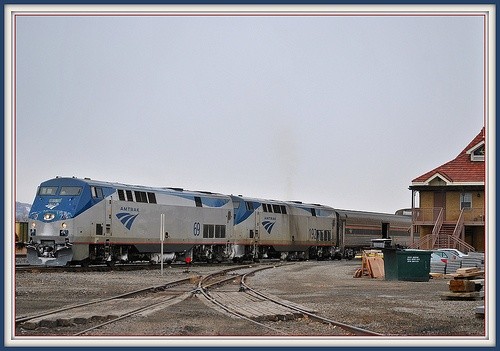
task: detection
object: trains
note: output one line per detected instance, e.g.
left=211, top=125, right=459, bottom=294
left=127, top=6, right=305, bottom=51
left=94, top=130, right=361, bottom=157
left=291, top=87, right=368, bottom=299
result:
left=24, top=176, right=421, bottom=271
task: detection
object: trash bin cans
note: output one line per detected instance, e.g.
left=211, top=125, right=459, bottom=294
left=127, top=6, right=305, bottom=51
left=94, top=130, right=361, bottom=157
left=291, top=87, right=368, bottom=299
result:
left=381, top=248, right=399, bottom=281
left=396, top=248, right=433, bottom=283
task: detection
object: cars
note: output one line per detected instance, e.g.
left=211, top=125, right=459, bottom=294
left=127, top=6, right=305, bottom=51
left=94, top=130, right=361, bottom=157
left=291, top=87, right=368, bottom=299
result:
left=404, top=247, right=468, bottom=261
